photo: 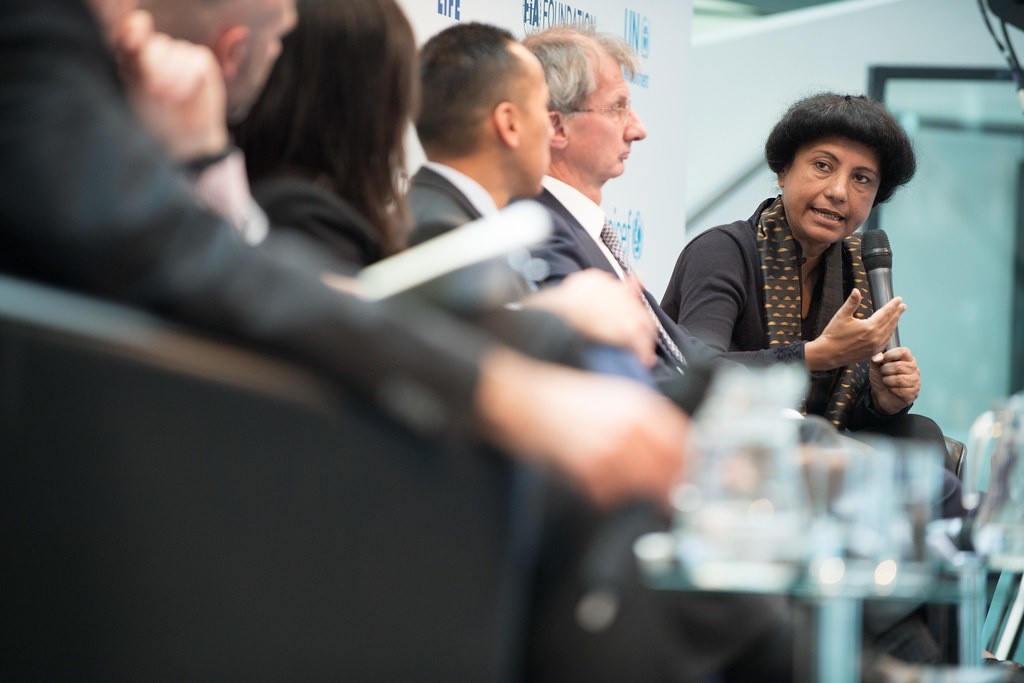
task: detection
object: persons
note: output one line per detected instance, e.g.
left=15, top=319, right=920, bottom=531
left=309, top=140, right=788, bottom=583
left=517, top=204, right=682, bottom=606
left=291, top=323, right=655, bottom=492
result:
left=650, top=90, right=952, bottom=469
left=0, top=0, right=1024, bottom=683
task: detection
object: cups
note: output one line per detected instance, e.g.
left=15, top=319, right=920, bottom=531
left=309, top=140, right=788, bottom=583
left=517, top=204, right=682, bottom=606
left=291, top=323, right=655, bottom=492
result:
left=670, top=362, right=947, bottom=590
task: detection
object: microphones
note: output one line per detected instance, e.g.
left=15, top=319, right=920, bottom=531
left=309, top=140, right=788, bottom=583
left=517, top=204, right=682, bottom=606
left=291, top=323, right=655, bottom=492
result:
left=860, top=229, right=900, bottom=353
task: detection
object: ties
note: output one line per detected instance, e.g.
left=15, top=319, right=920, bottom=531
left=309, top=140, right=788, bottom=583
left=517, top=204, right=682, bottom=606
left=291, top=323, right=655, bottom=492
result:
left=600, top=216, right=690, bottom=372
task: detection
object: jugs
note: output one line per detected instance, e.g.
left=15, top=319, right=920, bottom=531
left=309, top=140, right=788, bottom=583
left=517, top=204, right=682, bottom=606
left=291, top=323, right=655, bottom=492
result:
left=960, top=390, right=1024, bottom=569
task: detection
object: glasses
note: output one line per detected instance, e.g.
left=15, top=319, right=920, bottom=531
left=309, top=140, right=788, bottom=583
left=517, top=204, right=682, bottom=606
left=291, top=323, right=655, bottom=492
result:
left=571, top=103, right=630, bottom=124
